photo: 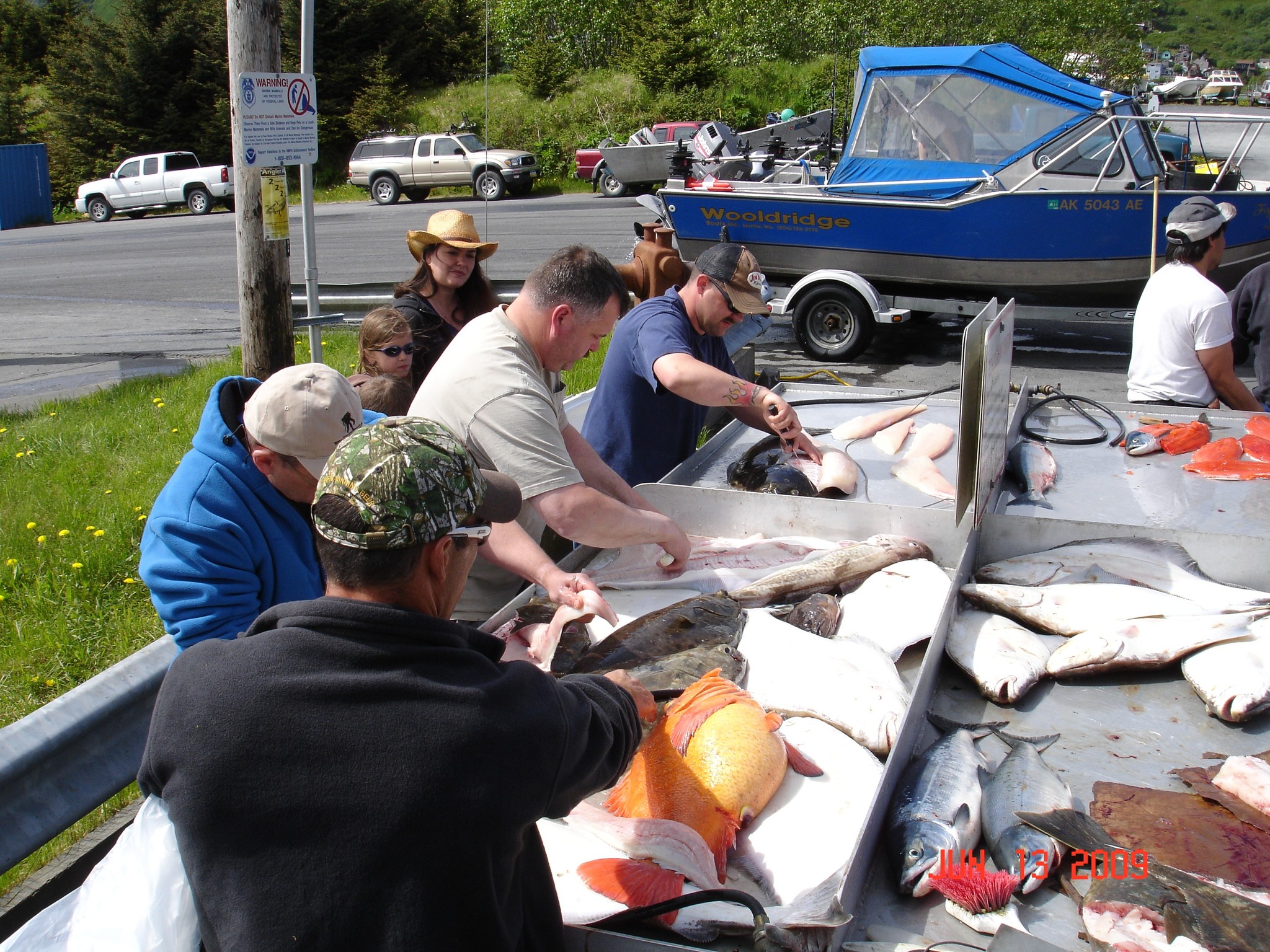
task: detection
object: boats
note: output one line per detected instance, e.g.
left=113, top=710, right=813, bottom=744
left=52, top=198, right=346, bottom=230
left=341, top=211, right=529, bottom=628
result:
left=655, top=14, right=1270, bottom=307
left=596, top=107, right=840, bottom=187
left=1152, top=70, right=1244, bottom=99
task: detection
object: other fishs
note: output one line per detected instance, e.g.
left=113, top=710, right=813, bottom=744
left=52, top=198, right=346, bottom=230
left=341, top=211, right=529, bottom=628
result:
left=495, top=404, right=1268, bottom=952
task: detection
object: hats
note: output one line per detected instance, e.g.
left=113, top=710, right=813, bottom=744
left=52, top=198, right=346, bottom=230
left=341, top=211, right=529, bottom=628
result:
left=1164, top=197, right=1237, bottom=244
left=243, top=363, right=367, bottom=481
left=406, top=209, right=499, bottom=264
left=695, top=243, right=771, bottom=316
left=310, top=416, right=523, bottom=549
left=874, top=86, right=902, bottom=114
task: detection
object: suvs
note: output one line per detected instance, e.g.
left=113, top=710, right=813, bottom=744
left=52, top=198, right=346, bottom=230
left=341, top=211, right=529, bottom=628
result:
left=348, top=127, right=543, bottom=205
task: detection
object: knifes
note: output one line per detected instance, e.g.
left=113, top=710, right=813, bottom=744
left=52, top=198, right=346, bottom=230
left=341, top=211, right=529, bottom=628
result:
left=769, top=405, right=806, bottom=471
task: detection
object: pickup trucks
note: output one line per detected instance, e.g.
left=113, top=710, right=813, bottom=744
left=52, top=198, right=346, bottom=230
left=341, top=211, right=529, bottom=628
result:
left=75, top=151, right=236, bottom=224
left=574, top=120, right=715, bottom=197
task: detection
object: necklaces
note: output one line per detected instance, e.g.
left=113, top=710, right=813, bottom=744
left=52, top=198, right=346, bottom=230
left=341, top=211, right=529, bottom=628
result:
left=423, top=284, right=453, bottom=325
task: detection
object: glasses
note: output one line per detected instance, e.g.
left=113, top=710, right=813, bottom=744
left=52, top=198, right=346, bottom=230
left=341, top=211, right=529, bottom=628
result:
left=707, top=276, right=742, bottom=314
left=447, top=520, right=492, bottom=546
left=370, top=341, right=416, bottom=357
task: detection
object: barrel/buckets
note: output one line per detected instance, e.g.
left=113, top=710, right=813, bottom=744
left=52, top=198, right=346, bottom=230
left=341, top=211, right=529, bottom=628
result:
left=1165, top=166, right=1240, bottom=191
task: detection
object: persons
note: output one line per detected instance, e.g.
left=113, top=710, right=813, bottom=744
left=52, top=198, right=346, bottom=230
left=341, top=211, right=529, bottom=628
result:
left=572, top=243, right=823, bottom=550
left=873, top=86, right=973, bottom=162
left=138, top=362, right=603, bottom=652
left=347, top=209, right=691, bottom=628
left=1127, top=196, right=1270, bottom=412
left=136, top=416, right=658, bottom=952
